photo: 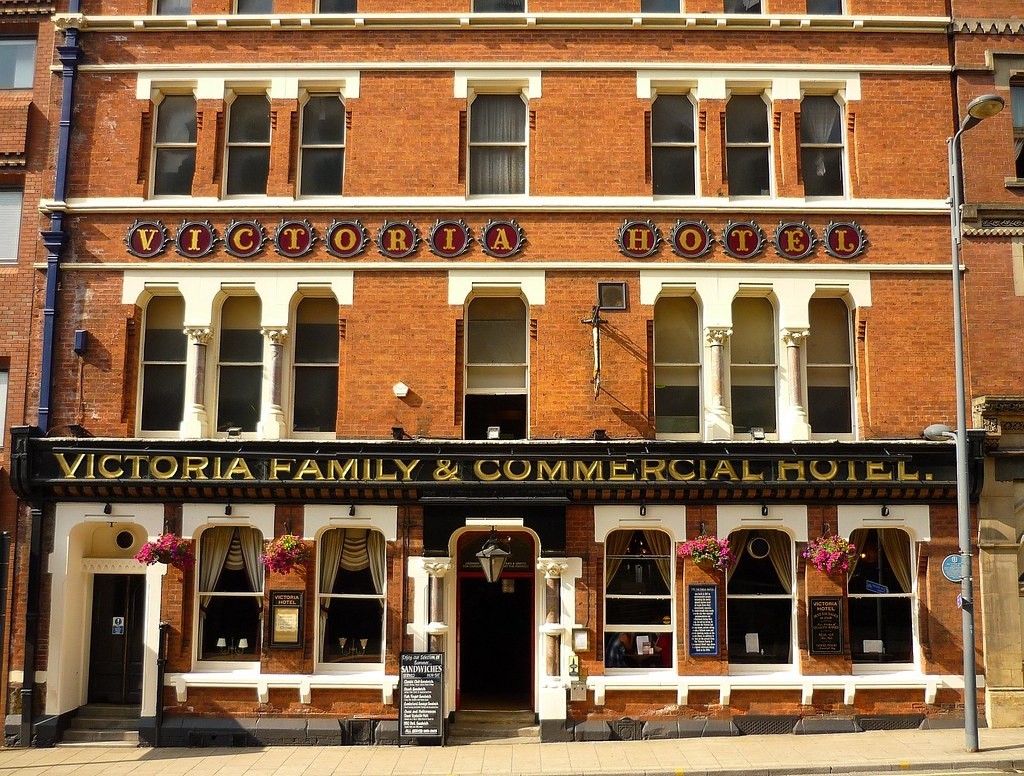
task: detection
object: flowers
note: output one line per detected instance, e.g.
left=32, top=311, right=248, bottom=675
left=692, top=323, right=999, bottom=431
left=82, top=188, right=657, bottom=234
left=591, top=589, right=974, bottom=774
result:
left=803, top=536, right=858, bottom=574
left=678, top=536, right=737, bottom=571
left=134, top=535, right=196, bottom=571
left=258, top=535, right=313, bottom=574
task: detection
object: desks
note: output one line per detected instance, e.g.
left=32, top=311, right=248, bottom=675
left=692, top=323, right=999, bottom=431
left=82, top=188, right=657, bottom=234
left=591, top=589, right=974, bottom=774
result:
left=856, top=652, right=893, bottom=661
left=624, top=653, right=661, bottom=667
left=731, top=654, right=777, bottom=662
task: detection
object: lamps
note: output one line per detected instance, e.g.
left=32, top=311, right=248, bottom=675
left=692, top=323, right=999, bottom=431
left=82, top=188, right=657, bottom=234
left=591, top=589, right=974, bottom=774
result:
left=348, top=503, right=356, bottom=516
left=750, top=427, right=765, bottom=441
left=476, top=525, right=511, bottom=584
left=763, top=503, right=768, bottom=516
left=216, top=636, right=248, bottom=659
left=338, top=637, right=368, bottom=661
left=639, top=501, right=647, bottom=516
left=882, top=501, right=889, bottom=515
left=227, top=426, right=242, bottom=440
left=592, top=428, right=607, bottom=439
left=487, top=426, right=500, bottom=440
left=104, top=500, right=113, bottom=514
left=224, top=502, right=232, bottom=515
left=391, top=426, right=412, bottom=442
left=67, top=424, right=87, bottom=438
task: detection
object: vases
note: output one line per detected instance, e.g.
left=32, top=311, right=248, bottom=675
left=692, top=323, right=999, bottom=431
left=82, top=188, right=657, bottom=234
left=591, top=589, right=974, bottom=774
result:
left=154, top=551, right=179, bottom=563
left=696, top=554, right=715, bottom=568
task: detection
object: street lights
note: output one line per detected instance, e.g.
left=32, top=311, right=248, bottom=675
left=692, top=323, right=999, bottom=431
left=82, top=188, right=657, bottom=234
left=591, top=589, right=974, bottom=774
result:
left=924, top=95, right=1006, bottom=753
